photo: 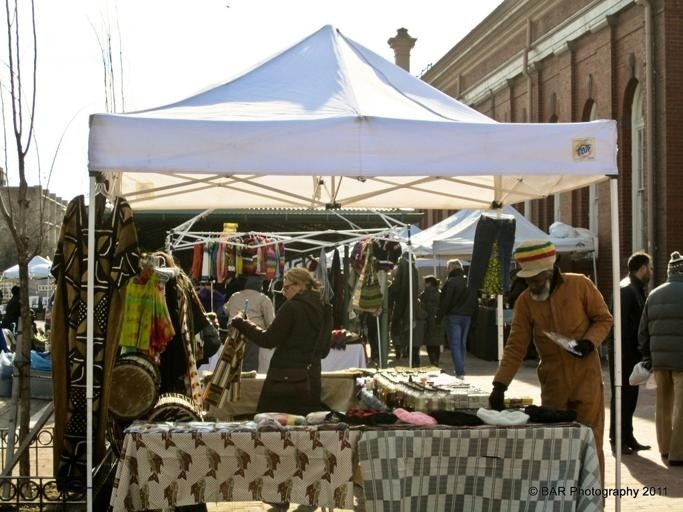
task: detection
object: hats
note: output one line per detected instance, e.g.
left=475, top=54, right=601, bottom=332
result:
left=667, top=252, right=683, bottom=276
left=514, top=241, right=555, bottom=278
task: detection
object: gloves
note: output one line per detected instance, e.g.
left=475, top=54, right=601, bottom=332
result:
left=489, top=386, right=505, bottom=411
left=567, top=340, right=593, bottom=358
left=231, top=316, right=242, bottom=330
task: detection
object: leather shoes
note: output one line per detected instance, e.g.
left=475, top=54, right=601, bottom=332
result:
left=611, top=436, right=649, bottom=455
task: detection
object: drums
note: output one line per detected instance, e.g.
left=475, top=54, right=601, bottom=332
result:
left=108, top=352, right=161, bottom=418
left=141, top=393, right=205, bottom=421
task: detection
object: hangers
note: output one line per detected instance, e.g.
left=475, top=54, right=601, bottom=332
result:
left=80, top=180, right=116, bottom=204
left=200, top=230, right=281, bottom=245
left=140, top=251, right=176, bottom=285
left=241, top=297, right=252, bottom=319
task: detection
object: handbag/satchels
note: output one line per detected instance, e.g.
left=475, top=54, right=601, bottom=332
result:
left=267, top=367, right=311, bottom=401
left=351, top=283, right=383, bottom=313
